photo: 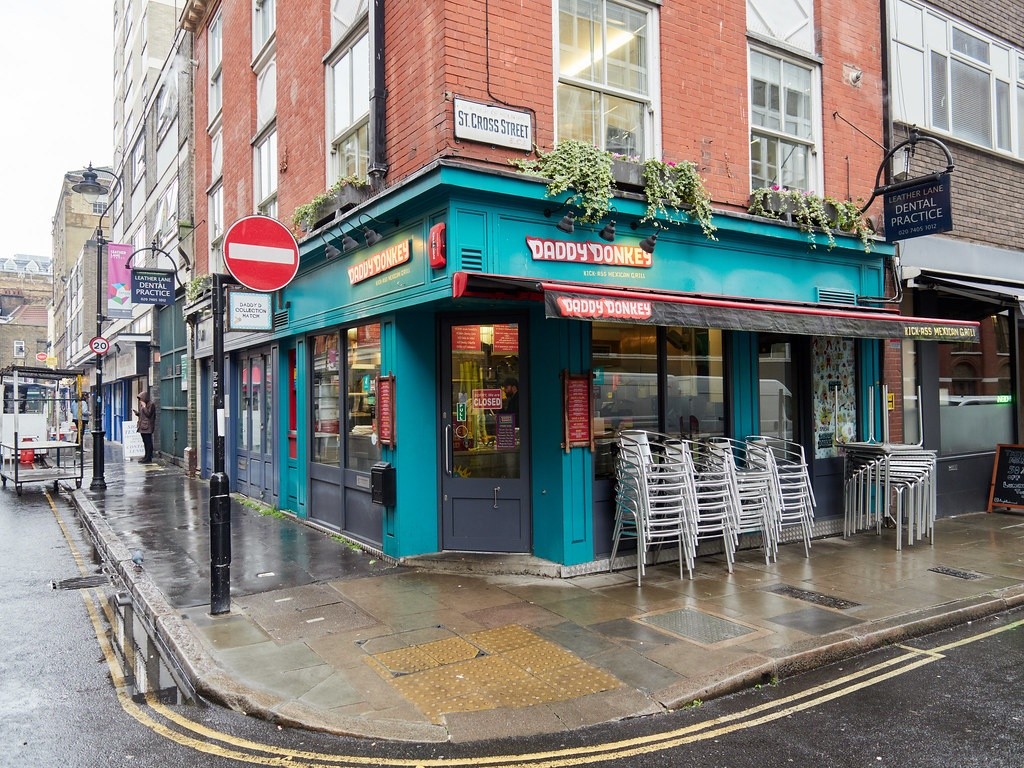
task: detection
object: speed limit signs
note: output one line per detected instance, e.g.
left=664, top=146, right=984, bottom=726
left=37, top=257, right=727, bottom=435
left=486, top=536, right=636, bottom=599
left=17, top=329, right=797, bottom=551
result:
left=91, top=337, right=111, bottom=354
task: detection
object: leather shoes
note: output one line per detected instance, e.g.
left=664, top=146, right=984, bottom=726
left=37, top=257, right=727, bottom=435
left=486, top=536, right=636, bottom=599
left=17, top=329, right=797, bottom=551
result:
left=138, top=456, right=152, bottom=463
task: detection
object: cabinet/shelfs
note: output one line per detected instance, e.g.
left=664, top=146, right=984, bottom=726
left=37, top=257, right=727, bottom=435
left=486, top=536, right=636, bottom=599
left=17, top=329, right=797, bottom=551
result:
left=0, top=365, right=85, bottom=496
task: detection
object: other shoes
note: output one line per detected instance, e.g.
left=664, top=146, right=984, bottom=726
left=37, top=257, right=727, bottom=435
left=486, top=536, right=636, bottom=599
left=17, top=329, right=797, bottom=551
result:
left=76, top=448, right=85, bottom=452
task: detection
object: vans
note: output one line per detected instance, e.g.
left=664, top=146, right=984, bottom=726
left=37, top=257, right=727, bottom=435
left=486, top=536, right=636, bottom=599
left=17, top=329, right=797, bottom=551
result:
left=677, top=375, right=792, bottom=441
left=589, top=372, right=676, bottom=431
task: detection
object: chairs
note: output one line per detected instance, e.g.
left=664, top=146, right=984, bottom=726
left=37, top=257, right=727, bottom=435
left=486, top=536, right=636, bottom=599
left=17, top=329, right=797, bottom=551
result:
left=744, top=435, right=817, bottom=563
left=701, top=436, right=781, bottom=566
left=609, top=429, right=704, bottom=587
left=654, top=439, right=743, bottom=573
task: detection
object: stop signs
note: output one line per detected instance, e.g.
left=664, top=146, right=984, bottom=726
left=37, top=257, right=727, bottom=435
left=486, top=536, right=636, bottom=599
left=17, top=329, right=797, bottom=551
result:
left=222, top=214, right=301, bottom=292
left=36, top=352, right=47, bottom=362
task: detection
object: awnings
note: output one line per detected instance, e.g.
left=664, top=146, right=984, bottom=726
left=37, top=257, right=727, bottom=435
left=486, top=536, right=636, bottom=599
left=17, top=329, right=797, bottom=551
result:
left=64, top=332, right=151, bottom=373
left=450, top=270, right=982, bottom=344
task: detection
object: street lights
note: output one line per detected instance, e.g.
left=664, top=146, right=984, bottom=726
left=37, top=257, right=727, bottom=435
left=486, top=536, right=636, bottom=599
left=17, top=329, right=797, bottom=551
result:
left=19, top=345, right=32, bottom=367
left=71, top=163, right=121, bottom=492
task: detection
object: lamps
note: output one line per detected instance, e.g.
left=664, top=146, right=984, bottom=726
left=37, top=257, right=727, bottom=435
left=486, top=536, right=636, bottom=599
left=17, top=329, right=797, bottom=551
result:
left=588, top=207, right=618, bottom=242
left=338, top=221, right=378, bottom=253
left=544, top=197, right=576, bottom=234
left=321, top=230, right=360, bottom=262
left=630, top=220, right=663, bottom=253
left=359, top=213, right=400, bottom=248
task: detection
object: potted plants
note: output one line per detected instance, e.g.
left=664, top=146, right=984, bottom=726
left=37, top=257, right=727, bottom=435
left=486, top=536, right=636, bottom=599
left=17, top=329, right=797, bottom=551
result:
left=750, top=187, right=873, bottom=254
left=292, top=176, right=363, bottom=233
left=512, top=141, right=719, bottom=244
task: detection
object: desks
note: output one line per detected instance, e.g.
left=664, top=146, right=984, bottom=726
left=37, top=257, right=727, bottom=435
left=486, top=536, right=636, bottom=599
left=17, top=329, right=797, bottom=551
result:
left=834, top=385, right=925, bottom=451
left=843, top=449, right=938, bottom=552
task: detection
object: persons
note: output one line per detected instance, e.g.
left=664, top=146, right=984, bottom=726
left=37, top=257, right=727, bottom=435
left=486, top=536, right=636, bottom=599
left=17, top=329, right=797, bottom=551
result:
left=69, top=391, right=93, bottom=452
left=496, top=377, right=519, bottom=428
left=132, top=391, right=156, bottom=464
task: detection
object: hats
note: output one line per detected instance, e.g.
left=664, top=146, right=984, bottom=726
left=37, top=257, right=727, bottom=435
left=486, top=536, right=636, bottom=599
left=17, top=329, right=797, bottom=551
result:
left=501, top=377, right=519, bottom=386
